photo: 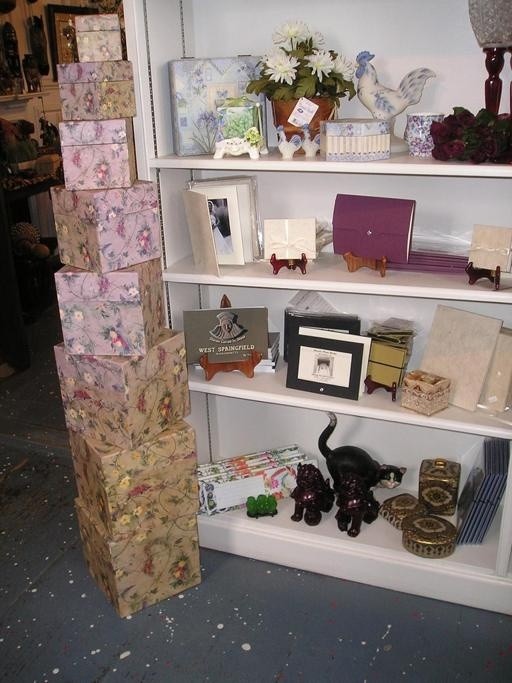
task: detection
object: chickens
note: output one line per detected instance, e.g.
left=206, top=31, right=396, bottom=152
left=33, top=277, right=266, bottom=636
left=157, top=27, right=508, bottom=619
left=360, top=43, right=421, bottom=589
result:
left=353, top=51, right=436, bottom=119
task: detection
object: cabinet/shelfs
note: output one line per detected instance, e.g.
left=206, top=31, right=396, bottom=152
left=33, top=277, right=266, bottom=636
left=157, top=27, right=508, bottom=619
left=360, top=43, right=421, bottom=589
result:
left=124, top=0, right=511, bottom=619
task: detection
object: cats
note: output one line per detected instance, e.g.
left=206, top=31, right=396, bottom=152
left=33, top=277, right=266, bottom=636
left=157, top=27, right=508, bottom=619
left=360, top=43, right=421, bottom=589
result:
left=317, top=411, right=407, bottom=491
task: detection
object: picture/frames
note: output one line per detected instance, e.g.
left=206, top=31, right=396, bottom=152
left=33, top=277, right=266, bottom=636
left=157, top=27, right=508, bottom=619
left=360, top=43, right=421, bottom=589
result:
left=281, top=309, right=374, bottom=403
left=47, top=1, right=101, bottom=81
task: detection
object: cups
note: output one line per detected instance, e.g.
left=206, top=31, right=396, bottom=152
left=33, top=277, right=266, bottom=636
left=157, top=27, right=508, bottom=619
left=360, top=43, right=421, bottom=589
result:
left=404, top=113, right=445, bottom=159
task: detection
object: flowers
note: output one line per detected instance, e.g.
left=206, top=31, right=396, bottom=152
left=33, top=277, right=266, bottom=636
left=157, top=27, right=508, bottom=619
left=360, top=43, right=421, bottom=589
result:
left=242, top=15, right=356, bottom=106
left=423, top=102, right=510, bottom=167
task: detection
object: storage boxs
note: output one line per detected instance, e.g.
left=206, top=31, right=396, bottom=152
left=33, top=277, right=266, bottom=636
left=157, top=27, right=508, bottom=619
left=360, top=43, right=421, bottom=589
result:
left=52, top=63, right=140, bottom=119
left=67, top=419, right=202, bottom=534
left=43, top=180, right=164, bottom=273
left=52, top=260, right=166, bottom=358
left=73, top=498, right=203, bottom=619
left=55, top=121, right=139, bottom=188
left=51, top=329, right=192, bottom=451
left=71, top=14, right=126, bottom=62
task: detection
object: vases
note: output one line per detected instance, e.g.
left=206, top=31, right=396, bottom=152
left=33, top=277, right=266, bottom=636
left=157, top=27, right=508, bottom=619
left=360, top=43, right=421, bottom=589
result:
left=270, top=98, right=334, bottom=144
